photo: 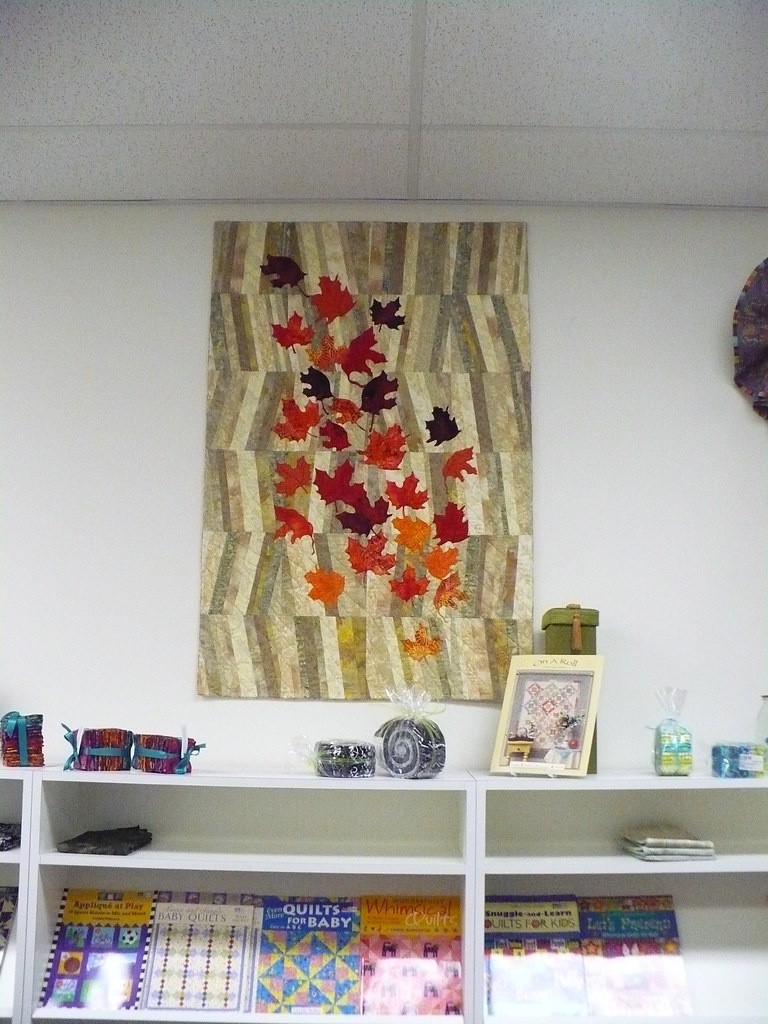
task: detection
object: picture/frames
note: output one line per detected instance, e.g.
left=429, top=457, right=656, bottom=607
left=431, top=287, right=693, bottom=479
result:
left=489, top=654, right=603, bottom=776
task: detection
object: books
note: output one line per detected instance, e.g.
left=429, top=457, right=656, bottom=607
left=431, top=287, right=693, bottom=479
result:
left=255, top=896, right=362, bottom=1014
left=578, top=894, right=692, bottom=1017
left=0, top=822, right=21, bottom=852
left=141, top=891, right=265, bottom=1013
left=58, top=826, right=153, bottom=856
left=483, top=892, right=588, bottom=1016
left=361, top=895, right=464, bottom=1016
left=0, top=886, right=19, bottom=967
left=38, top=888, right=159, bottom=1010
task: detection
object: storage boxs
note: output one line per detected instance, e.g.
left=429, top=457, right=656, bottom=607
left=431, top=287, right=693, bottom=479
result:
left=541, top=603, right=599, bottom=774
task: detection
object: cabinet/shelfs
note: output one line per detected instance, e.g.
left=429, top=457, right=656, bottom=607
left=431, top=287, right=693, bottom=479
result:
left=0, top=764, right=768, bottom=1024
